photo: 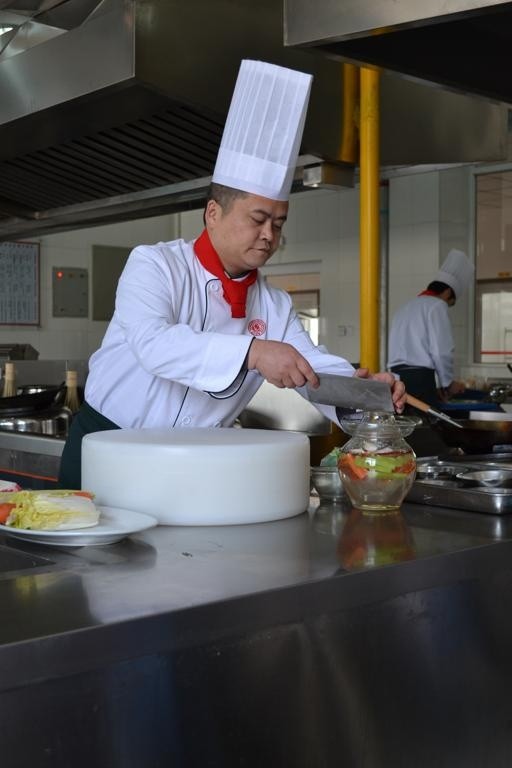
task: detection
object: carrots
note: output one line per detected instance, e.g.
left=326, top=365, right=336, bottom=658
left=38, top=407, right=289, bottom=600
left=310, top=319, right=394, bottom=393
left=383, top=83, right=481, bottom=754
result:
left=74, top=492, right=94, bottom=499
left=0, top=504, right=15, bottom=524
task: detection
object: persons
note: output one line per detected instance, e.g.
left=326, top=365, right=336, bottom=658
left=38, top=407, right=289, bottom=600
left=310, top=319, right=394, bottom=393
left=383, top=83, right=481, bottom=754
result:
left=389, top=249, right=468, bottom=415
left=57, top=60, right=405, bottom=490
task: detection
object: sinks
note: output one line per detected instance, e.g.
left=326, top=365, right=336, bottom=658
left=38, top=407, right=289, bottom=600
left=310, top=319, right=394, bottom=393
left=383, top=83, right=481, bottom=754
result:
left=0, top=406, right=73, bottom=440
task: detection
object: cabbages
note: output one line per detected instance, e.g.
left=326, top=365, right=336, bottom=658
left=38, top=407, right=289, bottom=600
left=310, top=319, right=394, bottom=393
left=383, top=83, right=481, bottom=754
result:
left=6, top=490, right=98, bottom=531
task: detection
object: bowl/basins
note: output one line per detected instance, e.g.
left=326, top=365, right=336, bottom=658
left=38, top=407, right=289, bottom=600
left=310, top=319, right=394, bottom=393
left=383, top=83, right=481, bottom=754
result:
left=417, top=466, right=511, bottom=493
left=313, top=468, right=349, bottom=503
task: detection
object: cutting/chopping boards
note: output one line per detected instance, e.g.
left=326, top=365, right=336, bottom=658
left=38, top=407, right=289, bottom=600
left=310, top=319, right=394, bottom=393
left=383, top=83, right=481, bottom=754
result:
left=81, top=429, right=311, bottom=526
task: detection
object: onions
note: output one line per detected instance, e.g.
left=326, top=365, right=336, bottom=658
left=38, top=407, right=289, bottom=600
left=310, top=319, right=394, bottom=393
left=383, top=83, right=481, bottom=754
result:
left=0, top=480, right=21, bottom=492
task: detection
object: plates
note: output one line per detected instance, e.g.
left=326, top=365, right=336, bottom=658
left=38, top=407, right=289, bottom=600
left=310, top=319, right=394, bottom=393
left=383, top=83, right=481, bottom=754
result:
left=0, top=506, right=159, bottom=547
left=405, top=462, right=512, bottom=515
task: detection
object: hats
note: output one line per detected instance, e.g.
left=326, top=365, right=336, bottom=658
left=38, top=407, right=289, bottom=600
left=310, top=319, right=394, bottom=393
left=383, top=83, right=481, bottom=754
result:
left=211, top=59, right=313, bottom=202
left=434, top=249, right=474, bottom=307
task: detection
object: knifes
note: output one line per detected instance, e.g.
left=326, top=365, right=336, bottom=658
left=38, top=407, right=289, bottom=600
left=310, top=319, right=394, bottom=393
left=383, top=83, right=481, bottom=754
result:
left=307, top=373, right=398, bottom=413
